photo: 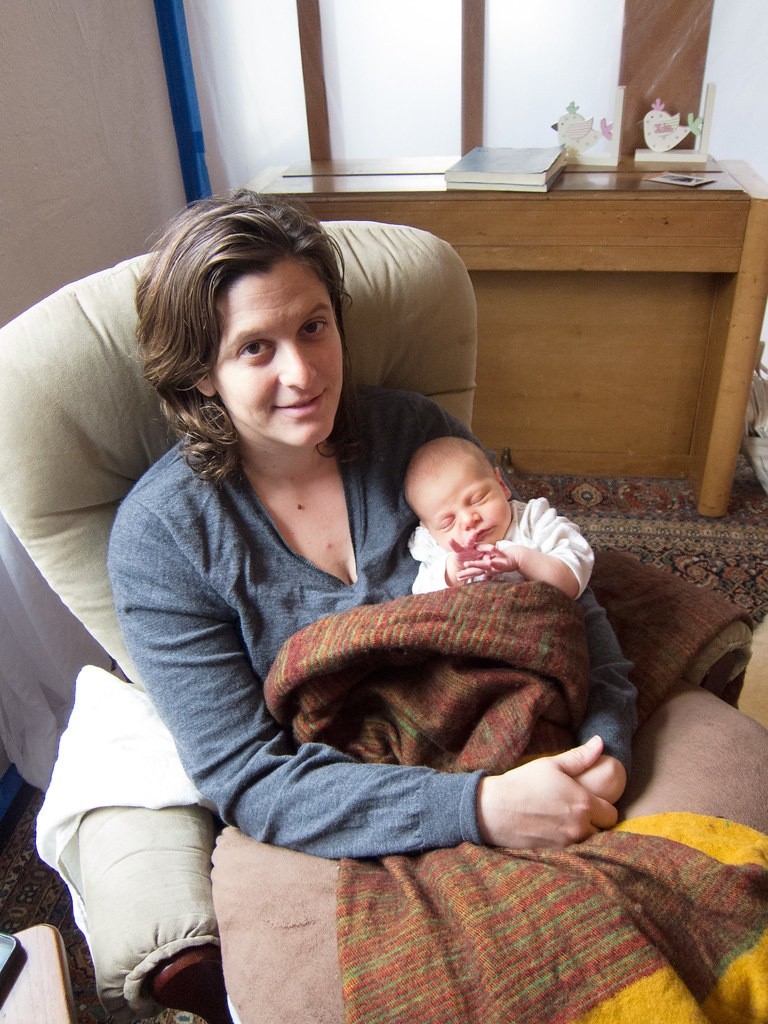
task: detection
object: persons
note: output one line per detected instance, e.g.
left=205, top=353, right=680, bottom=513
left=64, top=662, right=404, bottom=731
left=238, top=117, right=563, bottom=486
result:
left=109, top=188, right=640, bottom=855
left=396, top=434, right=594, bottom=603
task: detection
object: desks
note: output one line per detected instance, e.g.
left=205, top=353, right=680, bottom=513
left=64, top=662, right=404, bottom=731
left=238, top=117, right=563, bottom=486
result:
left=0, top=923, right=79, bottom=1024
left=240, top=154, right=768, bottom=518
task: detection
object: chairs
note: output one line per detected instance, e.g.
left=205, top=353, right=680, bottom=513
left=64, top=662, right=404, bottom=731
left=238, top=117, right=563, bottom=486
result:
left=0, top=219, right=755, bottom=1024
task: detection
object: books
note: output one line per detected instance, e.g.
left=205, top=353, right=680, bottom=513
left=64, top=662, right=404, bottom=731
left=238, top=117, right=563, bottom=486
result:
left=444, top=141, right=571, bottom=195
